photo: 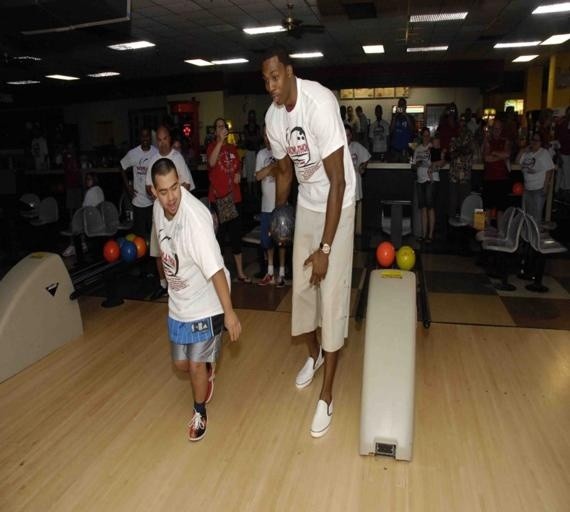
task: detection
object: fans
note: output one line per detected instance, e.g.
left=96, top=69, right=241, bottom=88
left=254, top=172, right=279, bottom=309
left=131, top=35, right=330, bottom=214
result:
left=267, top=2, right=328, bottom=40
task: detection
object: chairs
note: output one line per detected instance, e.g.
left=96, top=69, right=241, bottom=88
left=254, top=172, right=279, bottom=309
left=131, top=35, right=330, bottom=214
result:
left=473, top=203, right=569, bottom=295
left=9, top=185, right=292, bottom=275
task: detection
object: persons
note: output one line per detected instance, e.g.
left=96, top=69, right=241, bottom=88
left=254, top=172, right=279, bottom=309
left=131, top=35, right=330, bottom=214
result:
left=148, top=157, right=243, bottom=443
left=260, top=44, right=358, bottom=439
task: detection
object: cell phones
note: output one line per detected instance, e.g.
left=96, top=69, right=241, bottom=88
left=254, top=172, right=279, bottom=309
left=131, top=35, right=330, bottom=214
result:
left=224, top=124, right=228, bottom=130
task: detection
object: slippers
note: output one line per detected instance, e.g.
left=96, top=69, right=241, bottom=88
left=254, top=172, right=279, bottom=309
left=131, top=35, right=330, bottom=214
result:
left=418, top=236, right=426, bottom=241
left=425, top=238, right=434, bottom=245
left=238, top=275, right=252, bottom=284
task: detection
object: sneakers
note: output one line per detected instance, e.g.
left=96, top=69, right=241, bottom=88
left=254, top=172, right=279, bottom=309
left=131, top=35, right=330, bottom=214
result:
left=101, top=298, right=124, bottom=307
left=204, top=361, right=217, bottom=403
left=63, top=246, right=76, bottom=256
left=81, top=243, right=87, bottom=251
left=276, top=276, right=286, bottom=288
left=295, top=344, right=325, bottom=389
left=310, top=397, right=334, bottom=439
left=257, top=273, right=275, bottom=286
left=188, top=407, right=207, bottom=441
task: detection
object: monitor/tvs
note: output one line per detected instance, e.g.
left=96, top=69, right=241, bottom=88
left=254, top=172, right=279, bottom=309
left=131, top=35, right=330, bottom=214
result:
left=19, top=1, right=132, bottom=35
